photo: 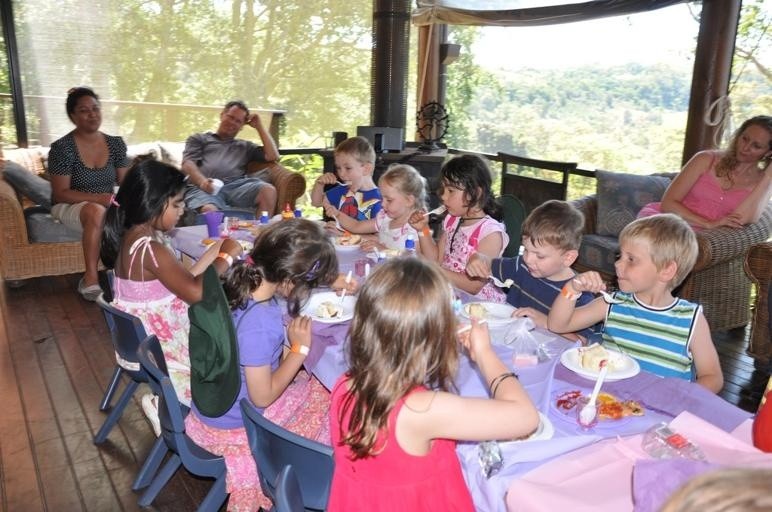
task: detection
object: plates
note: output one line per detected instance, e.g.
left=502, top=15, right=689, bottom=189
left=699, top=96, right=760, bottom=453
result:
left=560, top=347, right=641, bottom=382
left=237, top=227, right=255, bottom=230
left=366, top=250, right=402, bottom=262
left=236, top=239, right=255, bottom=254
left=331, top=237, right=369, bottom=251
left=550, top=388, right=630, bottom=428
left=461, top=300, right=520, bottom=326
left=499, top=411, right=554, bottom=444
left=295, top=292, right=359, bottom=324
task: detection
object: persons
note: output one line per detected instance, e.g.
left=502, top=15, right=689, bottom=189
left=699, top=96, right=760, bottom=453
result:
left=181, top=216, right=333, bottom=512
left=179, top=99, right=280, bottom=221
left=48, top=86, right=129, bottom=303
left=324, top=255, right=540, bottom=511
left=636, top=114, right=772, bottom=237
left=658, top=466, right=772, bottom=512
left=99, top=158, right=244, bottom=441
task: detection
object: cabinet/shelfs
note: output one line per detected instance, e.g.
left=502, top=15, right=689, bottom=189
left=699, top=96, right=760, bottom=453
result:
left=315, top=141, right=448, bottom=229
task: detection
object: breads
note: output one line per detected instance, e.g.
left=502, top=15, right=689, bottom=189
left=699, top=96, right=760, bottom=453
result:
left=317, top=301, right=337, bottom=317
left=582, top=345, right=611, bottom=371
left=469, top=303, right=483, bottom=317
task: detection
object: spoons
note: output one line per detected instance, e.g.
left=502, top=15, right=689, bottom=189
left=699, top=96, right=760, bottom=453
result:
left=580, top=367, right=608, bottom=425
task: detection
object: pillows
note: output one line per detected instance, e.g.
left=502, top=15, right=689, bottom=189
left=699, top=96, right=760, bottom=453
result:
left=2, top=156, right=54, bottom=210
left=158, top=141, right=187, bottom=170
left=588, top=166, right=674, bottom=240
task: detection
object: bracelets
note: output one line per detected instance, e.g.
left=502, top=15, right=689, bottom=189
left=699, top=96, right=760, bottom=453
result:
left=289, top=343, right=309, bottom=357
left=215, top=252, right=234, bottom=268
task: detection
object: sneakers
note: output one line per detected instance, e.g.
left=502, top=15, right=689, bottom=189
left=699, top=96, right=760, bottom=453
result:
left=77, top=275, right=104, bottom=302
left=140, top=391, right=166, bottom=439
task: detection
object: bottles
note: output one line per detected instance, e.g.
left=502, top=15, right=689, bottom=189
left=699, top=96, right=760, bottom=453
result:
left=640, top=421, right=711, bottom=464
left=281, top=201, right=303, bottom=219
left=753, top=375, right=772, bottom=454
left=378, top=252, right=387, bottom=264
left=260, top=211, right=269, bottom=226
left=403, top=234, right=415, bottom=251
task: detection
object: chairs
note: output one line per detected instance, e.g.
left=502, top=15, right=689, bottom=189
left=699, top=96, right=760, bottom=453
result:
left=569, top=161, right=770, bottom=342
left=89, top=290, right=155, bottom=447
left=234, top=394, right=339, bottom=512
left=130, top=330, right=233, bottom=512
left=490, top=193, right=526, bottom=255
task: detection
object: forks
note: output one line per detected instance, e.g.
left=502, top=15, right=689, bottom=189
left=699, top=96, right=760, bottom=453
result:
left=332, top=270, right=353, bottom=317
left=317, top=179, right=351, bottom=186
left=573, top=279, right=625, bottom=305
left=331, top=214, right=346, bottom=232
left=423, top=205, right=448, bottom=216
left=486, top=273, right=509, bottom=288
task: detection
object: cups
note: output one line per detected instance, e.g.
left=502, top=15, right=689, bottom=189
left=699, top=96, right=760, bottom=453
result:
left=353, top=258, right=371, bottom=281
left=224, top=217, right=240, bottom=232
left=211, top=178, right=224, bottom=196
left=326, top=137, right=334, bottom=150
left=332, top=131, right=348, bottom=148
left=575, top=396, right=601, bottom=433
left=374, top=133, right=383, bottom=152
left=204, top=210, right=224, bottom=238
left=216, top=222, right=233, bottom=238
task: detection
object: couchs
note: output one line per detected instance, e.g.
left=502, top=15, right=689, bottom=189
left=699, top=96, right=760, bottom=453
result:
left=737, top=240, right=770, bottom=375
left=2, top=138, right=306, bottom=290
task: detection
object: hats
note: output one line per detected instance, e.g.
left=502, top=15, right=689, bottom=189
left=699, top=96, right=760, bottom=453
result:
left=188, top=261, right=243, bottom=419
